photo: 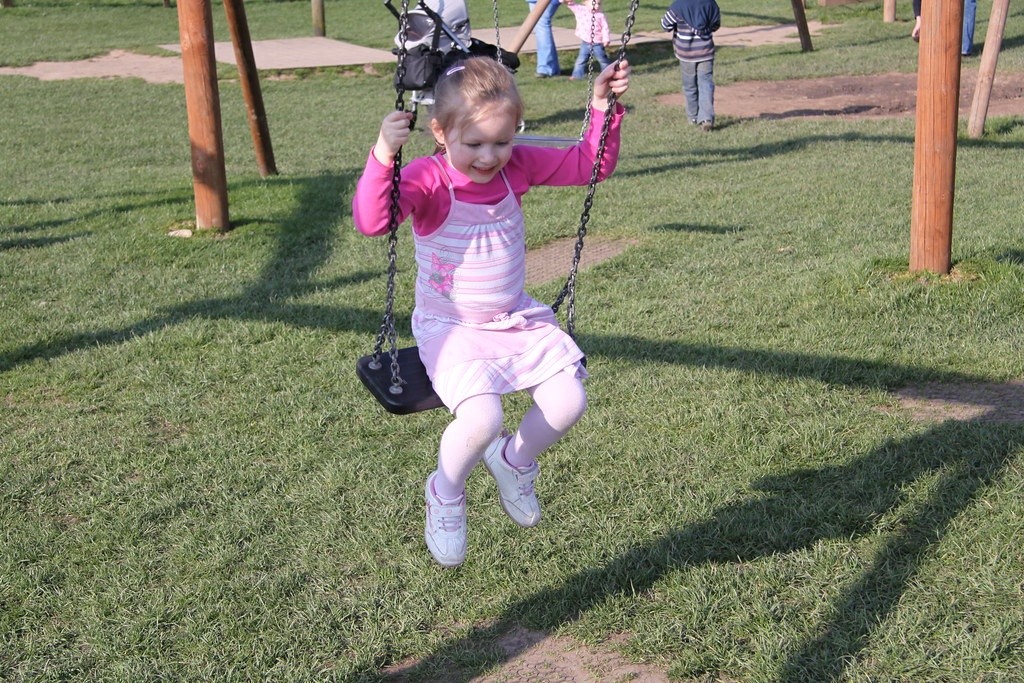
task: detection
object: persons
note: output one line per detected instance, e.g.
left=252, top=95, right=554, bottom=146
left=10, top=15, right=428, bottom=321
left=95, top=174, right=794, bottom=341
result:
left=393, top=0, right=520, bottom=105
left=661, top=0, right=721, bottom=131
left=912, top=0, right=977, bottom=56
left=526, top=0, right=611, bottom=80
left=352, top=56, right=632, bottom=566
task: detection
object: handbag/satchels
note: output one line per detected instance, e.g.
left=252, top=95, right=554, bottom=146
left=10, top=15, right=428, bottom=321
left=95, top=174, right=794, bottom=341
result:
left=393, top=13, right=444, bottom=90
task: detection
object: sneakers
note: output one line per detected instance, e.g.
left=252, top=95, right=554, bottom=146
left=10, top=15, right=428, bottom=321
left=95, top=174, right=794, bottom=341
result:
left=482, top=427, right=541, bottom=527
left=425, top=471, right=468, bottom=566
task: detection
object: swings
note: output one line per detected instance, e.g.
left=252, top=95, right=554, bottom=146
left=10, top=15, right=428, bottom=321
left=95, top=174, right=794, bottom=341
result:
left=354, top=0, right=644, bottom=420
left=491, top=0, right=596, bottom=146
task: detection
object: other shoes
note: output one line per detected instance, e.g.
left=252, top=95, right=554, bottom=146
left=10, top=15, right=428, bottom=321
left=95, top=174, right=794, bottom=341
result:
left=702, top=120, right=712, bottom=131
left=536, top=73, right=550, bottom=79
